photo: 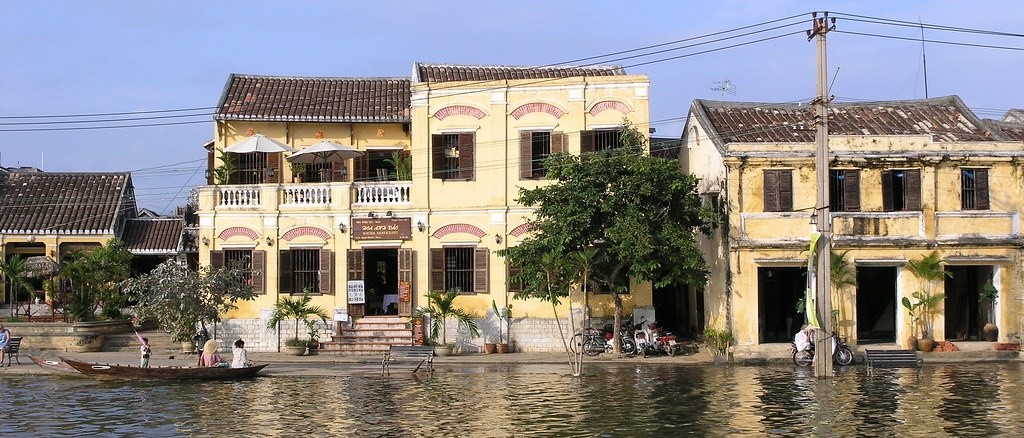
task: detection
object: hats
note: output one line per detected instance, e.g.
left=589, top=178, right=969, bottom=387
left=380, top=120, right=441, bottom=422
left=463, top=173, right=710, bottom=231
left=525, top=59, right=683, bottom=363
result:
left=204, top=338, right=218, bottom=355
left=199, top=326, right=207, bottom=334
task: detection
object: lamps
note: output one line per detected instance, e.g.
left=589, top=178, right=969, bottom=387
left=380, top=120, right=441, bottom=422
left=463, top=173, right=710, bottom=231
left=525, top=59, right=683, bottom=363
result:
left=339, top=222, right=345, bottom=232
left=341, top=165, right=347, bottom=176
left=495, top=233, right=501, bottom=243
left=367, top=211, right=394, bottom=219
left=201, top=235, right=208, bottom=246
left=417, top=220, right=423, bottom=232
left=205, top=169, right=210, bottom=179
left=266, top=237, right=272, bottom=245
left=268, top=167, right=274, bottom=177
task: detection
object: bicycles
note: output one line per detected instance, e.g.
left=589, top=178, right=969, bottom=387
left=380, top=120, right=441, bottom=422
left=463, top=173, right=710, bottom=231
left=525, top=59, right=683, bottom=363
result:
left=791, top=328, right=854, bottom=366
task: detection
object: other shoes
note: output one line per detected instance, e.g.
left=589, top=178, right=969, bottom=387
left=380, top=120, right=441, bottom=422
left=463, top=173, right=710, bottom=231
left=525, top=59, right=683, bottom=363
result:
left=0, top=361, right=5, bottom=367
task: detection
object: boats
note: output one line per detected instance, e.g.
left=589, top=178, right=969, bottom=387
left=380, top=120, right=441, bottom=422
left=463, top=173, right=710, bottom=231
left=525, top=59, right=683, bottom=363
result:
left=24, top=351, right=90, bottom=380
left=56, top=353, right=271, bottom=380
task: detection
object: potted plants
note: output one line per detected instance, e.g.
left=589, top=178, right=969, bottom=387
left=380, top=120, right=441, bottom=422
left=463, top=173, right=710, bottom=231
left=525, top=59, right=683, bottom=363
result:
left=491, top=299, right=512, bottom=353
left=899, top=250, right=954, bottom=352
left=901, top=295, right=921, bottom=352
left=267, top=287, right=330, bottom=355
left=977, top=280, right=999, bottom=341
left=289, top=162, right=305, bottom=183
left=304, top=317, right=320, bottom=353
left=409, top=288, right=480, bottom=357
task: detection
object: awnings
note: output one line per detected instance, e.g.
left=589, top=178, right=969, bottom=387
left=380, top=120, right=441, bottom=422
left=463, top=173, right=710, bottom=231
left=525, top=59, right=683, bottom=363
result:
left=204, top=139, right=215, bottom=155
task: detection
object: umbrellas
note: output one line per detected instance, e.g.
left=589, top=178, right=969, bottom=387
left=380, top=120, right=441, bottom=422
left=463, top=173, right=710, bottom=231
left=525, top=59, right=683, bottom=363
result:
left=286, top=139, right=364, bottom=182
left=223, top=134, right=294, bottom=184
left=134, top=330, right=150, bottom=350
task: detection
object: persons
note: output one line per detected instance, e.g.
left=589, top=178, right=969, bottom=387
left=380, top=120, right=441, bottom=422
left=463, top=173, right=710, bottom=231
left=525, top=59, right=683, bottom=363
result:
left=192, top=327, right=210, bottom=366
left=0, top=323, right=11, bottom=363
left=232, top=340, right=258, bottom=368
left=201, top=340, right=230, bottom=368
left=140, top=338, right=151, bottom=367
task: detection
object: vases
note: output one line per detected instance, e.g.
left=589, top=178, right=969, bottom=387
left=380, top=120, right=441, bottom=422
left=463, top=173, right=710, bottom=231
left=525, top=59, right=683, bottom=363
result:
left=481, top=339, right=495, bottom=355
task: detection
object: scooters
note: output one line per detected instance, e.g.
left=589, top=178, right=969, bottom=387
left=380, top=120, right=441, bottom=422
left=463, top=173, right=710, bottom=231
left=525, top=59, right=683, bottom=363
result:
left=568, top=315, right=678, bottom=358
